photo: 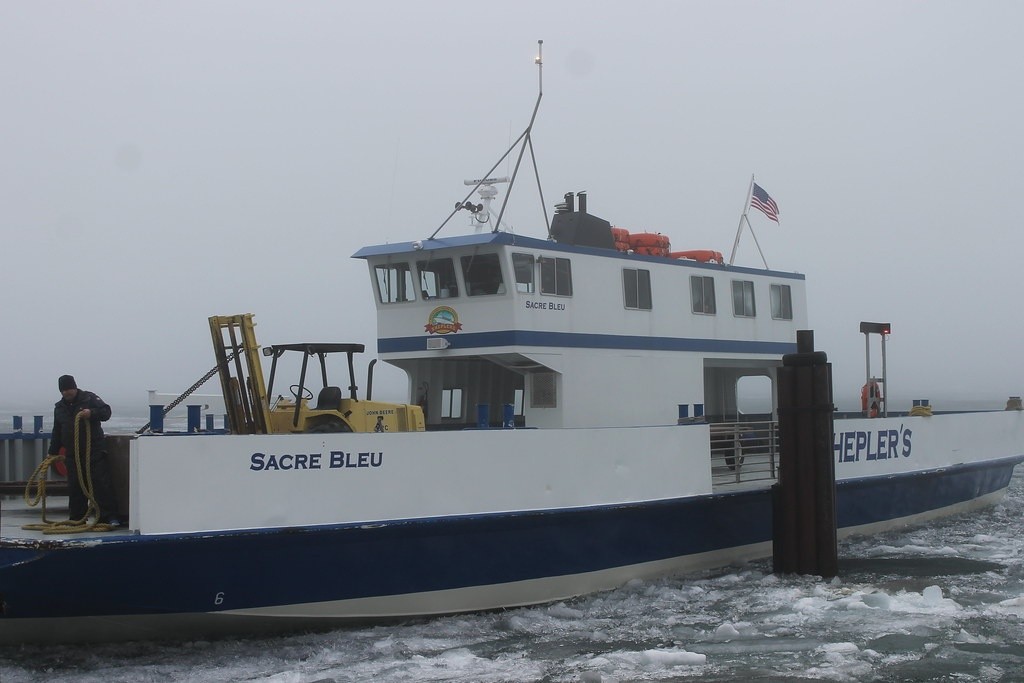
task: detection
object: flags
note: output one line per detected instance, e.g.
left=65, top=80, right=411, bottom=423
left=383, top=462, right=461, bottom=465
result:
left=751, top=182, right=779, bottom=224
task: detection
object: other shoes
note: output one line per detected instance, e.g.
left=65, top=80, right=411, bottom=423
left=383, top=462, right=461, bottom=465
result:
left=108, top=519, right=120, bottom=527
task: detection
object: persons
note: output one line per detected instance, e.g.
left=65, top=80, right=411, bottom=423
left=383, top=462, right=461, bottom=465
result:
left=46, top=374, right=121, bottom=529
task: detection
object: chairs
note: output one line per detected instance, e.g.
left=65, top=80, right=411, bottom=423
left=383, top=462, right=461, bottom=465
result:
left=311, top=386, right=342, bottom=411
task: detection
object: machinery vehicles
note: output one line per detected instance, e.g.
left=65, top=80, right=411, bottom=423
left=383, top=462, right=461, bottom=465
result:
left=130, top=311, right=426, bottom=437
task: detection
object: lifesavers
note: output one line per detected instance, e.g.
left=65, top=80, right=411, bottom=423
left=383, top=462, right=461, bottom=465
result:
left=725, top=435, right=745, bottom=469
left=611, top=227, right=670, bottom=256
left=54, top=446, right=68, bottom=476
left=860, top=381, right=881, bottom=417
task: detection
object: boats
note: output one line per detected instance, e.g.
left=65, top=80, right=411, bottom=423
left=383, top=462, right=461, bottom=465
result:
left=1, top=35, right=1024, bottom=649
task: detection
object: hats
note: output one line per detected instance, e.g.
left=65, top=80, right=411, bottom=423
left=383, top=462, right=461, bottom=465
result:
left=59, top=374, right=77, bottom=392
